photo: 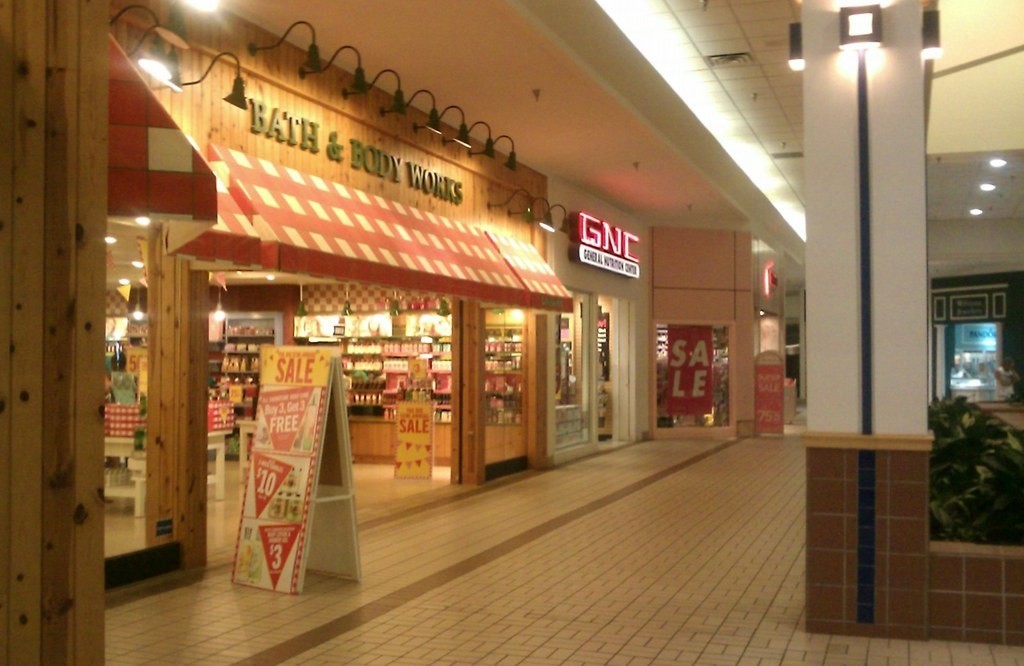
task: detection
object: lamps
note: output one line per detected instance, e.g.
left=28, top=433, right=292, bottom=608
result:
left=299, top=45, right=369, bottom=96
left=441, top=121, right=495, bottom=159
left=342, top=69, right=407, bottom=116
left=789, top=22, right=803, bottom=71
left=249, top=21, right=323, bottom=73
left=108, top=4, right=173, bottom=81
left=380, top=89, right=443, bottom=136
left=126, top=25, right=184, bottom=94
left=181, top=51, right=249, bottom=112
left=839, top=4, right=883, bottom=50
left=486, top=188, right=535, bottom=223
left=527, top=203, right=573, bottom=236
left=133, top=285, right=227, bottom=322
left=340, top=284, right=505, bottom=317
left=413, top=105, right=472, bottom=149
left=923, top=10, right=942, bottom=60
left=507, top=196, right=556, bottom=233
left=468, top=134, right=518, bottom=172
left=154, top=0, right=192, bottom=50
left=296, top=281, right=309, bottom=317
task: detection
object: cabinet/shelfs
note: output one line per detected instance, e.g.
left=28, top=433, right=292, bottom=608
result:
left=209, top=335, right=275, bottom=407
left=342, top=308, right=526, bottom=467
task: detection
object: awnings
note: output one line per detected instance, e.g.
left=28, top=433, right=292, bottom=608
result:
left=95, top=31, right=220, bottom=255
left=172, top=139, right=575, bottom=318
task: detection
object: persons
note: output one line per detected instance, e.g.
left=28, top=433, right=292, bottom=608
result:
left=104, top=350, right=139, bottom=468
left=951, top=357, right=1021, bottom=402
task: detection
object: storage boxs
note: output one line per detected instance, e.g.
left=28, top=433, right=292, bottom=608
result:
left=207, top=400, right=234, bottom=432
left=248, top=343, right=258, bottom=352
left=555, top=404, right=583, bottom=445
left=237, top=343, right=247, bottom=352
left=229, top=386, right=243, bottom=403
left=224, top=344, right=236, bottom=352
left=105, top=404, right=147, bottom=438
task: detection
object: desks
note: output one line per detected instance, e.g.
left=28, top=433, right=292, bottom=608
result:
left=236, top=420, right=256, bottom=485
left=104, top=430, right=232, bottom=518
